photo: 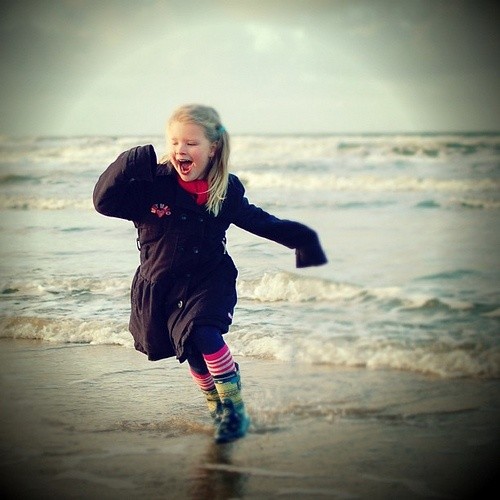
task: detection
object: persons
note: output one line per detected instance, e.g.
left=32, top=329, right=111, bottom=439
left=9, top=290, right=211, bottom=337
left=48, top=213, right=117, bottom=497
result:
left=93, top=104, right=328, bottom=445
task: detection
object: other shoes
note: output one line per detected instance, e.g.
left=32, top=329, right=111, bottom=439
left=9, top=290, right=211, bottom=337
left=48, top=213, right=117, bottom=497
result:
left=213, top=404, right=250, bottom=443
left=209, top=401, right=223, bottom=422
